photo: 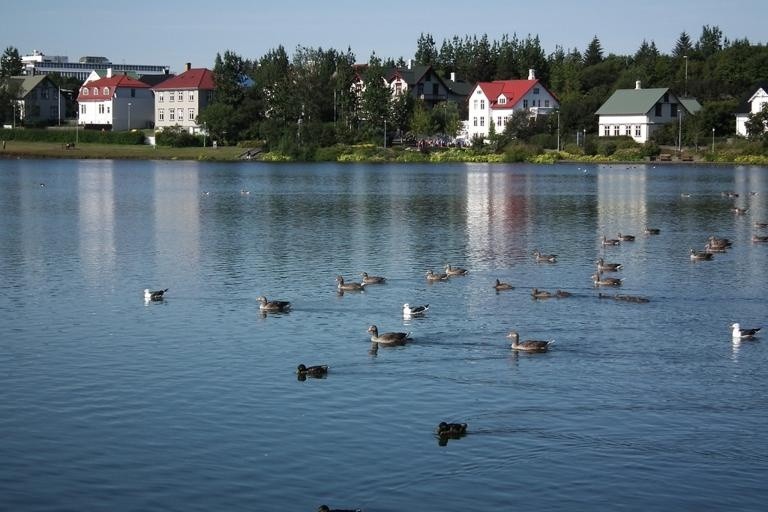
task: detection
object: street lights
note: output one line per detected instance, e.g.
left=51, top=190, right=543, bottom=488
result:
left=73, top=112, right=81, bottom=144
left=682, top=55, right=687, bottom=98
left=711, top=128, right=715, bottom=151
left=555, top=110, right=560, bottom=152
left=13, top=104, right=16, bottom=128
left=676, top=109, right=682, bottom=151
left=383, top=120, right=388, bottom=148
left=126, top=102, right=131, bottom=131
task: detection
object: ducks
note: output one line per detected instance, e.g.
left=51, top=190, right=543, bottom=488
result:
left=143, top=189, right=767, bottom=512
